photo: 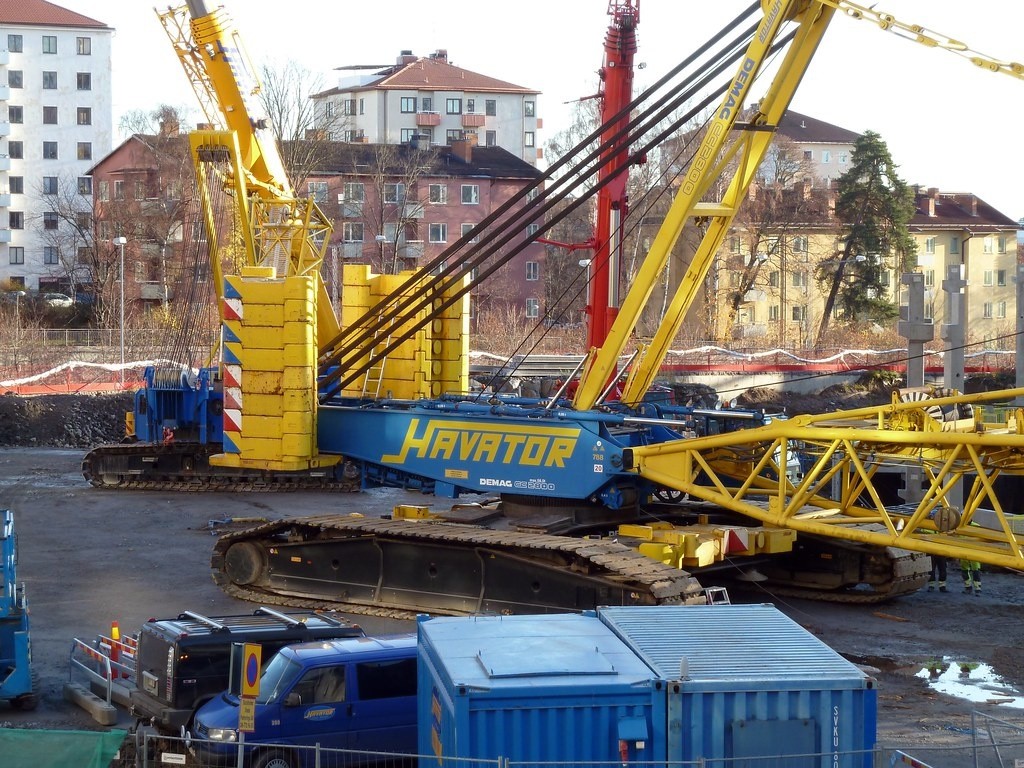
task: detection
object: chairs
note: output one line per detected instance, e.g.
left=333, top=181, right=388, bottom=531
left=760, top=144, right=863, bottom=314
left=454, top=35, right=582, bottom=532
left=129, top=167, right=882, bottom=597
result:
left=311, top=672, right=339, bottom=702
left=329, top=681, right=347, bottom=702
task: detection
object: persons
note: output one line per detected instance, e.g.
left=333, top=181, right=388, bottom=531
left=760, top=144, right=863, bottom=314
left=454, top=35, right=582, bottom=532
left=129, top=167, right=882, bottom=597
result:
left=920, top=509, right=952, bottom=593
left=955, top=509, right=983, bottom=598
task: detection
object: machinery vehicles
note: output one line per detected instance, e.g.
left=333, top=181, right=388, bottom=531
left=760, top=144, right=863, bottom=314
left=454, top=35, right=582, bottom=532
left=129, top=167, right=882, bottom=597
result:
left=80, top=0, right=1024, bottom=620
left=0, top=508, right=33, bottom=709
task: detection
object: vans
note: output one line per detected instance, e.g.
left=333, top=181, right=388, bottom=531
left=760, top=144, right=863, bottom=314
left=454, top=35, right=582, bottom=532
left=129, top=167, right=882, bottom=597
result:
left=183, top=634, right=417, bottom=768
left=128, top=608, right=366, bottom=736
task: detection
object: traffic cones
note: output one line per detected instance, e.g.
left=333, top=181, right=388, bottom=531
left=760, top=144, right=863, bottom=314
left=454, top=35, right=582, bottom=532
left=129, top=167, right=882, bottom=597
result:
left=102, top=620, right=128, bottom=679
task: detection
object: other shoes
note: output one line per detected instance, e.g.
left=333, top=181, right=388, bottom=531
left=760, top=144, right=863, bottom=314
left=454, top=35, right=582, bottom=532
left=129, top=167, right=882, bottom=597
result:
left=940, top=586, right=951, bottom=593
left=928, top=586, right=935, bottom=593
left=962, top=586, right=973, bottom=595
left=975, top=591, right=981, bottom=596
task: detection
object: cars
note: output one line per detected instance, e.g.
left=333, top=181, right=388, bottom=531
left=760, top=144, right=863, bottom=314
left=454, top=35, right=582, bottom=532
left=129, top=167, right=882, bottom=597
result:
left=44, top=293, right=72, bottom=307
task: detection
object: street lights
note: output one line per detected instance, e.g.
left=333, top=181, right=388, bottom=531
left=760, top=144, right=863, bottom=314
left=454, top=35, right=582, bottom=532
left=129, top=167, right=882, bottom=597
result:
left=15, top=291, right=26, bottom=347
